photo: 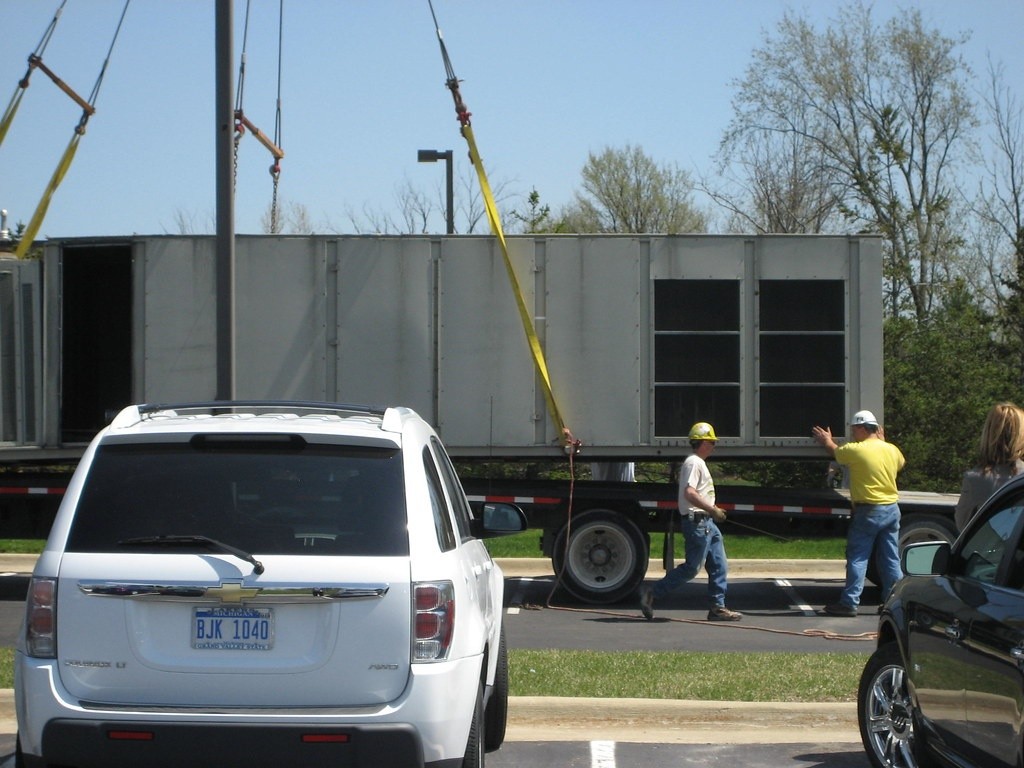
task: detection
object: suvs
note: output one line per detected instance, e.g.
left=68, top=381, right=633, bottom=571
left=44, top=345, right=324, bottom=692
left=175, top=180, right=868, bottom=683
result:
left=15, top=399, right=526, bottom=768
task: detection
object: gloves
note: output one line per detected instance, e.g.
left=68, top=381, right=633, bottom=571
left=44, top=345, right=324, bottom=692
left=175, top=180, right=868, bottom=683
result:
left=710, top=505, right=726, bottom=523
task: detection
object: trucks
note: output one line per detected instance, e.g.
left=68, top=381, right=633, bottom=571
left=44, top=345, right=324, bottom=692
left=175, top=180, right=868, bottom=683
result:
left=1, top=210, right=961, bottom=610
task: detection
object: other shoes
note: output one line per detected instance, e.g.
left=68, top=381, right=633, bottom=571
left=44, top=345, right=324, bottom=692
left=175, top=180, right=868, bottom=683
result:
left=823, top=604, right=858, bottom=617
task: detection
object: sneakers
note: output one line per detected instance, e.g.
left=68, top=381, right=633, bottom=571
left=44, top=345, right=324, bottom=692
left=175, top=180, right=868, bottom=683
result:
left=638, top=583, right=656, bottom=621
left=707, top=608, right=741, bottom=621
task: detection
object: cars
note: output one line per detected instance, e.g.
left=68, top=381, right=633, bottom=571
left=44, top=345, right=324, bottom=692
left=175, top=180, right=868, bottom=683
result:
left=856, top=473, right=1024, bottom=768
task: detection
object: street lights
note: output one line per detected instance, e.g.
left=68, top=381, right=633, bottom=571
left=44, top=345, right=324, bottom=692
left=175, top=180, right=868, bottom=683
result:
left=417, top=150, right=454, bottom=235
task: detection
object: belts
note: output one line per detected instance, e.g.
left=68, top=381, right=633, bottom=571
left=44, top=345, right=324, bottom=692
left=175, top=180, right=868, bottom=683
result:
left=682, top=513, right=712, bottom=519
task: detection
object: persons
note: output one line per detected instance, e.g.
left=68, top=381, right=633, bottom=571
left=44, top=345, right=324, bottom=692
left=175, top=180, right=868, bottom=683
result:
left=812, top=410, right=905, bottom=617
left=636, top=422, right=743, bottom=621
left=954, top=402, right=1024, bottom=533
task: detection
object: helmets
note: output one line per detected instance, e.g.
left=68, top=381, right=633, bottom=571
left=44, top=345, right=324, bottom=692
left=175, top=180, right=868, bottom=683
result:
left=850, top=410, right=879, bottom=426
left=689, top=422, right=719, bottom=441
